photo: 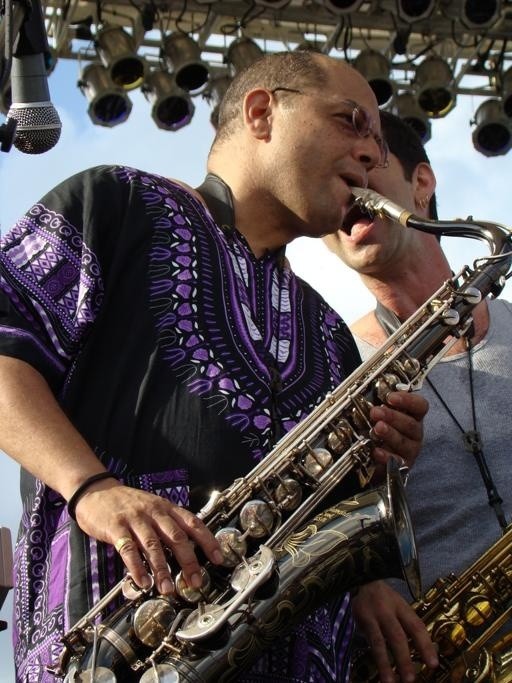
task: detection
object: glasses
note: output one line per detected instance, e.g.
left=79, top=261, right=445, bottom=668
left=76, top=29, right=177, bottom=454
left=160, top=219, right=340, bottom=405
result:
left=271, top=87, right=389, bottom=169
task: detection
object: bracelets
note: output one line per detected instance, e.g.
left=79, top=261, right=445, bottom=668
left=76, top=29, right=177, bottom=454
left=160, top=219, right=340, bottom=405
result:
left=67, top=471, right=120, bottom=520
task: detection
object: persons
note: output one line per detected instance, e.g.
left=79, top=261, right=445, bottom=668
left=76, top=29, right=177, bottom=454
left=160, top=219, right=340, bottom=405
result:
left=319, top=110, right=511, bottom=680
left=1, top=49, right=430, bottom=682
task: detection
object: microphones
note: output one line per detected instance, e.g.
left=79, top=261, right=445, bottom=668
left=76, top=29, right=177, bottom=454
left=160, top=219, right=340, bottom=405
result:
left=1, top=0, right=65, bottom=154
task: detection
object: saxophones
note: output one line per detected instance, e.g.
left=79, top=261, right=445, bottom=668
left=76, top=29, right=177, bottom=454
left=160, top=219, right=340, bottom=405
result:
left=48, top=183, right=511, bottom=682
left=356, top=524, right=511, bottom=683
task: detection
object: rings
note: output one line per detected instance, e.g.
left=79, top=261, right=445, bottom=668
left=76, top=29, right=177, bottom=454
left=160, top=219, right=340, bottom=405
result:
left=114, top=537, right=133, bottom=554
